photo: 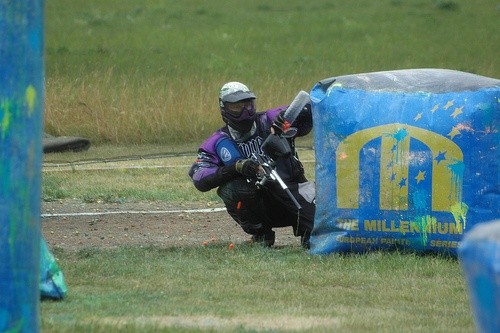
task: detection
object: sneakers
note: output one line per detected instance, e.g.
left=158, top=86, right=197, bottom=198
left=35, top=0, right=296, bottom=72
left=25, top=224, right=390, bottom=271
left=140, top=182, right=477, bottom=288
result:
left=249, top=232, right=275, bottom=248
left=302, top=237, right=310, bottom=249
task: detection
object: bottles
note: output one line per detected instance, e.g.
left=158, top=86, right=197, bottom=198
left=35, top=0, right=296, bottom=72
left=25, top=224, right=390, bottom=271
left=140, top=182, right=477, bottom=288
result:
left=271, top=90, right=310, bottom=136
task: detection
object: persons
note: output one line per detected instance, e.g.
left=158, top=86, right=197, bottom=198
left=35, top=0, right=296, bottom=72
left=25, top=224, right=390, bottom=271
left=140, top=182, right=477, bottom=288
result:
left=188, top=82, right=317, bottom=248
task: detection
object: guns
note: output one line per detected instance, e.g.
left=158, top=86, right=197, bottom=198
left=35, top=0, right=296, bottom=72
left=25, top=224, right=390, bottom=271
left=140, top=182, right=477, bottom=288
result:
left=213, top=132, right=302, bottom=211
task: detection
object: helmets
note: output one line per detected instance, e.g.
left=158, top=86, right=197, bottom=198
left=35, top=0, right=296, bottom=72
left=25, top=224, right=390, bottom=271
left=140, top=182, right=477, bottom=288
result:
left=218, top=81, right=257, bottom=133
